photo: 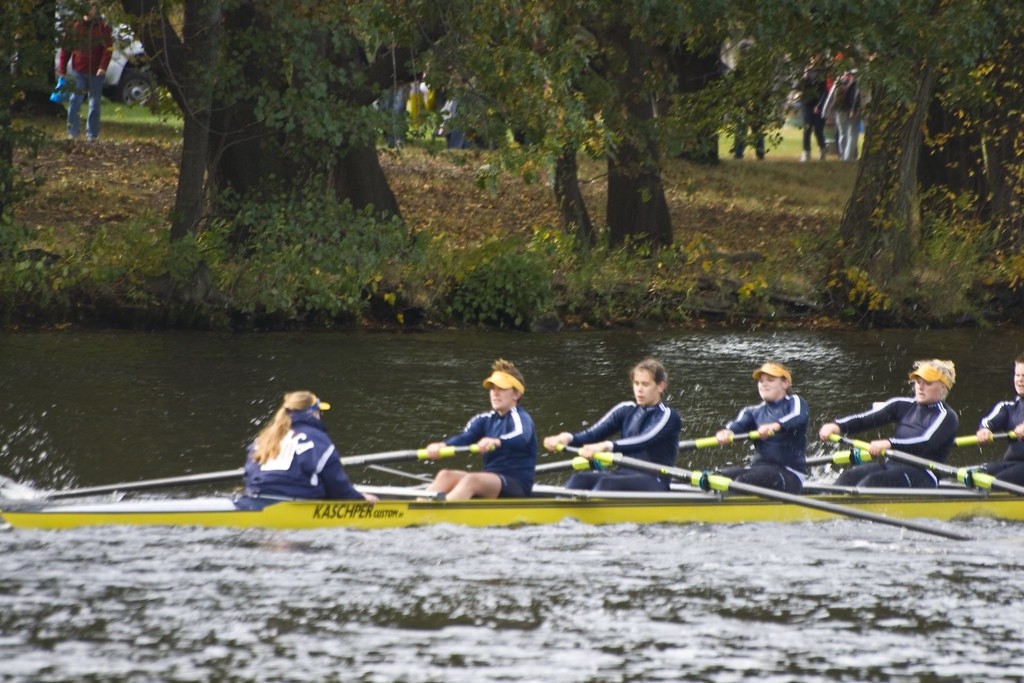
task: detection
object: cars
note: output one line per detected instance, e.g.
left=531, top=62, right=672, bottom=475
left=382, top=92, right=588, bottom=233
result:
left=54, top=11, right=159, bottom=107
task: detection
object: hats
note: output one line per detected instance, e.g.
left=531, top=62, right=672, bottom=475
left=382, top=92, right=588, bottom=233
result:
left=753, top=363, right=791, bottom=384
left=483, top=370, right=525, bottom=395
left=285, top=394, right=332, bottom=424
left=910, top=366, right=953, bottom=390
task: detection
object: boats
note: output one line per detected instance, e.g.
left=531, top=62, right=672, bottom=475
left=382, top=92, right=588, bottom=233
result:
left=0, top=477, right=1024, bottom=527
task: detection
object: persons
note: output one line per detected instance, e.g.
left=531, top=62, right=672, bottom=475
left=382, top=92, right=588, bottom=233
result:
left=411, top=358, right=539, bottom=501
left=376, top=37, right=874, bottom=164
left=716, top=361, right=808, bottom=497
left=977, top=354, right=1024, bottom=490
left=233, top=391, right=379, bottom=511
left=819, top=360, right=960, bottom=494
left=57, top=0, right=113, bottom=141
left=543, top=358, right=681, bottom=500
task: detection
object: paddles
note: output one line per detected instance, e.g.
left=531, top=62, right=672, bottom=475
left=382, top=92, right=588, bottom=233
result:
left=557, top=444, right=971, bottom=542
left=828, top=434, right=1023, bottom=498
left=805, top=431, right=1017, bottom=467
left=44, top=442, right=497, bottom=500
left=531, top=428, right=776, bottom=472
